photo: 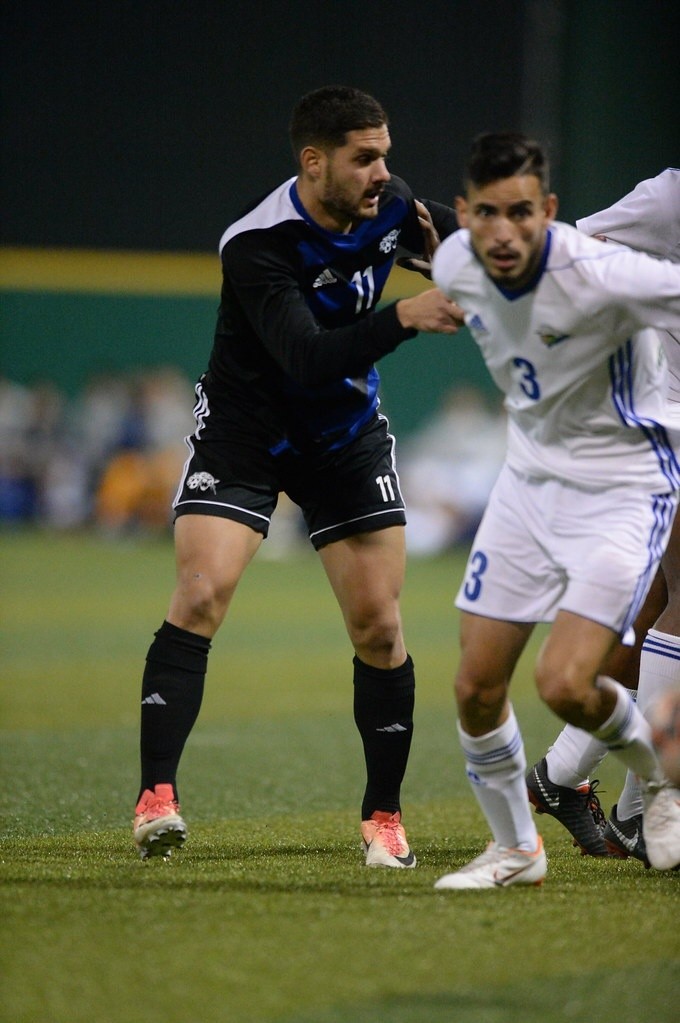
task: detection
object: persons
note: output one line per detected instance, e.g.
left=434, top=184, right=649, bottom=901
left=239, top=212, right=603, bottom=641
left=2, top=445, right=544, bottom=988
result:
left=0, top=370, right=510, bottom=570
left=431, top=132, right=680, bottom=889
left=135, top=88, right=464, bottom=869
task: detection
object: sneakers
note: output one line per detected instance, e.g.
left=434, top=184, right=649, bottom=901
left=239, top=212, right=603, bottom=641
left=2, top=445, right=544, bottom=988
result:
left=434, top=835, right=546, bottom=890
left=133, top=783, right=187, bottom=862
left=601, top=804, right=680, bottom=875
left=360, top=810, right=417, bottom=868
left=635, top=774, right=680, bottom=871
left=526, top=757, right=628, bottom=860
